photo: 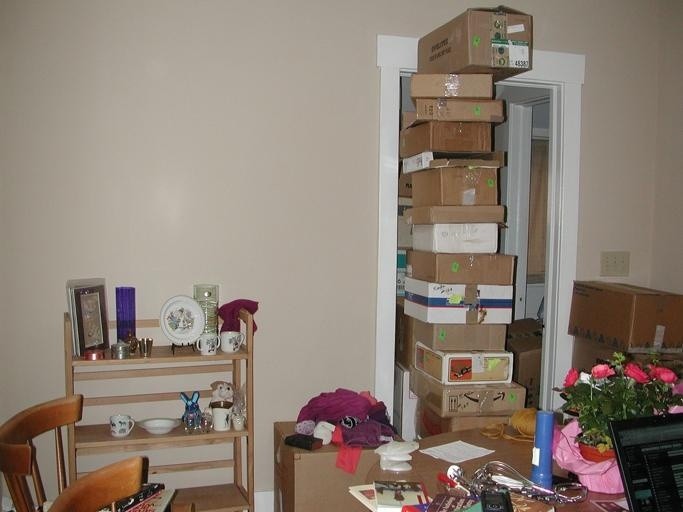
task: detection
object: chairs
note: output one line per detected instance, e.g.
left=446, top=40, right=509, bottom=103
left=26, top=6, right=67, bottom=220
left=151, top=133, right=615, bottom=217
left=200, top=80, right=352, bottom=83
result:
left=47, top=452, right=147, bottom=511
left=0, top=392, right=89, bottom=511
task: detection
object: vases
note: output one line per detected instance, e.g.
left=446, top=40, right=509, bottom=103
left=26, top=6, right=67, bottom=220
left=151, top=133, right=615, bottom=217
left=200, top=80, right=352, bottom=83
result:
left=578, top=442, right=617, bottom=464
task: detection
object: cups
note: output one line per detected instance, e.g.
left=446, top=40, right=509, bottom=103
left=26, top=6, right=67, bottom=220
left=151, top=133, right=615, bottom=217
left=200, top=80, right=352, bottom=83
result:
left=139, top=338, right=153, bottom=357
left=197, top=331, right=244, bottom=356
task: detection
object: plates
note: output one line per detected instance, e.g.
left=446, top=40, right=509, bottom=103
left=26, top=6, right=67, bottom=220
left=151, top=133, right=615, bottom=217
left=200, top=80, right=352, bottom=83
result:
left=160, top=296, right=205, bottom=347
left=136, top=419, right=181, bottom=434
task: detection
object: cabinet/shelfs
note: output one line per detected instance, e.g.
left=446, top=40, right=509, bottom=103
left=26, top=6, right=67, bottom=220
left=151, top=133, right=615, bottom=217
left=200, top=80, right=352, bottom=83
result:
left=63, top=311, right=256, bottom=512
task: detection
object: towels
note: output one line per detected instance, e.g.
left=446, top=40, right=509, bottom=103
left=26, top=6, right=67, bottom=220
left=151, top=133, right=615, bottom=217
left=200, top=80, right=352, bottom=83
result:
left=216, top=297, right=258, bottom=335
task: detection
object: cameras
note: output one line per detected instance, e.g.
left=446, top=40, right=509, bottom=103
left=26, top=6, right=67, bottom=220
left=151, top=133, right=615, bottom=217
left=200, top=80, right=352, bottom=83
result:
left=482, top=487, right=513, bottom=512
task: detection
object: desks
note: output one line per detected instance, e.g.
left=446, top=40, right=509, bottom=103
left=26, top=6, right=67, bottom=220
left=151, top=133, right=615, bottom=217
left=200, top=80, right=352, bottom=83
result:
left=359, top=427, right=632, bottom=512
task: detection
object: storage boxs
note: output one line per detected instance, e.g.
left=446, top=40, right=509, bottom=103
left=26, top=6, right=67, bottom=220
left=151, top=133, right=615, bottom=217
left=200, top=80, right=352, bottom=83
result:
left=570, top=338, right=683, bottom=376
left=269, top=417, right=406, bottom=512
left=568, top=280, right=683, bottom=357
left=403, top=4, right=546, bottom=437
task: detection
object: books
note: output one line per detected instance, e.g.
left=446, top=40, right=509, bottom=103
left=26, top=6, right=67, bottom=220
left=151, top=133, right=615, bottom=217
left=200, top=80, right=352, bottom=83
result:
left=348, top=479, right=555, bottom=512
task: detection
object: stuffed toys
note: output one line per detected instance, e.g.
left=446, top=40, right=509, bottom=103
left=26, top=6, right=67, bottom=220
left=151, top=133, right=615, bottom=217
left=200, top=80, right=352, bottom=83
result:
left=179, top=391, right=202, bottom=431
left=204, top=380, right=235, bottom=420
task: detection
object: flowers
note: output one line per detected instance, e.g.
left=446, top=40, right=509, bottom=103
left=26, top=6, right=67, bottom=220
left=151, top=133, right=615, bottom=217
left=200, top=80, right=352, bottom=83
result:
left=550, top=351, right=682, bottom=451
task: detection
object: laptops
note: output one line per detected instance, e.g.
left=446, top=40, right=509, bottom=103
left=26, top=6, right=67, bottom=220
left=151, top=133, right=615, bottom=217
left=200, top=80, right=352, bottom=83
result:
left=608, top=412, right=683, bottom=512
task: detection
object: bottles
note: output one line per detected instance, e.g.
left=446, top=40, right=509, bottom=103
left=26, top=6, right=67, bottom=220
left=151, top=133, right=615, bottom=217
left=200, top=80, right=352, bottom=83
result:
left=84, top=329, right=139, bottom=361
left=110, top=413, right=134, bottom=438
left=201, top=408, right=245, bottom=431
left=194, top=284, right=218, bottom=334
